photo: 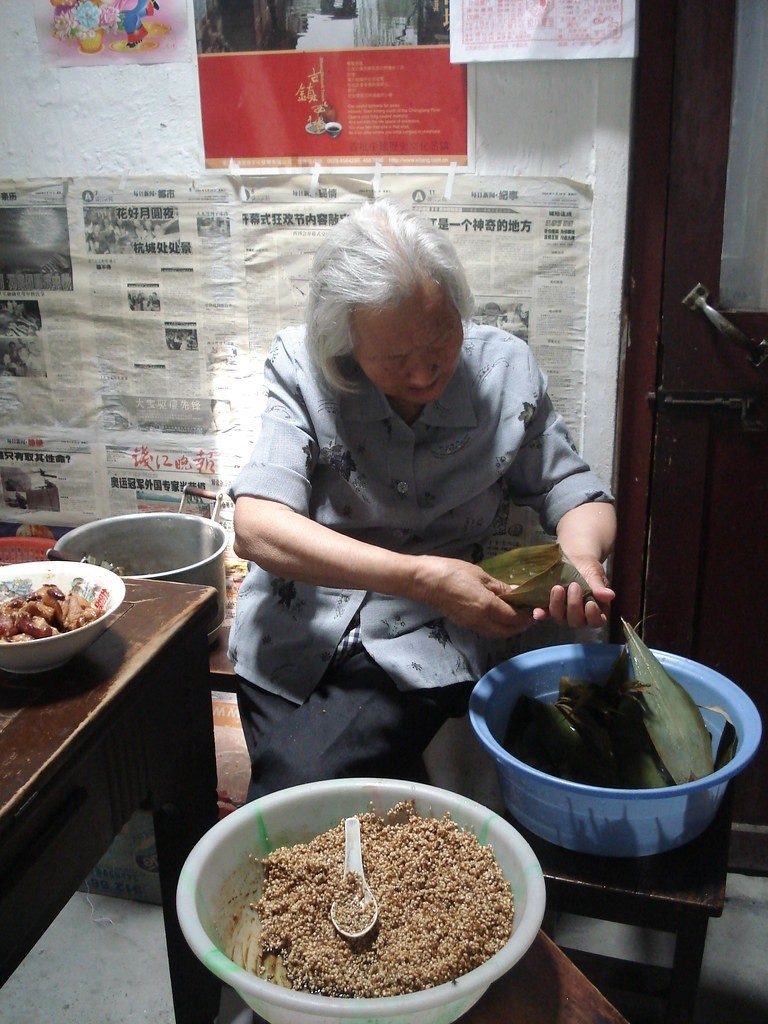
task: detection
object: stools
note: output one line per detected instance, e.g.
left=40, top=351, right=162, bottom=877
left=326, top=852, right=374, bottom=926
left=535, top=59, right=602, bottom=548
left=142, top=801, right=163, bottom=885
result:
left=453, top=928, right=631, bottom=1024
left=505, top=778, right=736, bottom=1024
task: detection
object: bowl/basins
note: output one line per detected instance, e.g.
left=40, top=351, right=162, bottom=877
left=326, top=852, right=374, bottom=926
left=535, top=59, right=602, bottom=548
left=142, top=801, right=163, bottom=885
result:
left=469, top=643, right=762, bottom=857
left=176, top=777, right=546, bottom=1024
left=0, top=561, right=126, bottom=674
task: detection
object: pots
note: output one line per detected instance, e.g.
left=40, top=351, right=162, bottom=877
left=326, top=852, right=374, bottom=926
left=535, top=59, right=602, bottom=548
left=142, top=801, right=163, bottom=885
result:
left=46, top=484, right=228, bottom=644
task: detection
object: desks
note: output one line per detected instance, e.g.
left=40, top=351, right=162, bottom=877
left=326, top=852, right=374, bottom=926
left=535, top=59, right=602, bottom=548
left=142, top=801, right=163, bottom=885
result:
left=0, top=581, right=220, bottom=1024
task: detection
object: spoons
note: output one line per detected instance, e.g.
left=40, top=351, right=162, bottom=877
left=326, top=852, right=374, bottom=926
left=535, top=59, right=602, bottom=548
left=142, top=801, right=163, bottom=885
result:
left=330, top=818, right=378, bottom=937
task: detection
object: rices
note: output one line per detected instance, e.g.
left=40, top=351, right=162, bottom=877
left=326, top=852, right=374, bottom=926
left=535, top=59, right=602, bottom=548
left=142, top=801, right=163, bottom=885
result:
left=247, top=802, right=515, bottom=998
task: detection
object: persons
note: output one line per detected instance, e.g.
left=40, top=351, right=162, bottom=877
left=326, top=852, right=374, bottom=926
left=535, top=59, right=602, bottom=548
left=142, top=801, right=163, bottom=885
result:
left=128, top=292, right=160, bottom=311
left=1, top=341, right=46, bottom=377
left=85, top=215, right=175, bottom=254
left=226, top=198, right=618, bottom=1024
left=479, top=299, right=503, bottom=330
